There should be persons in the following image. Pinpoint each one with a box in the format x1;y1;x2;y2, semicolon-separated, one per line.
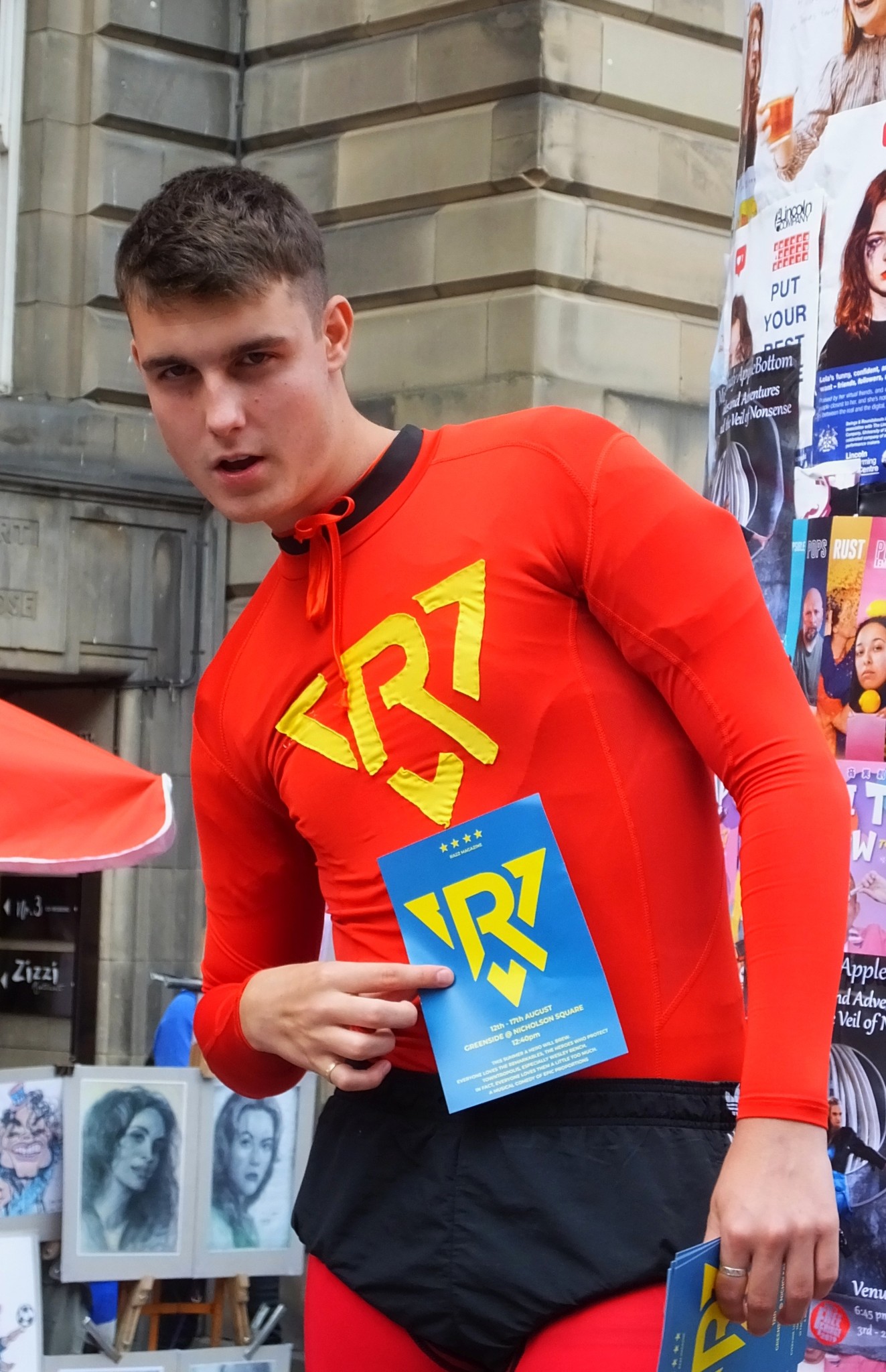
731;0;886;1372
115;166;853;1372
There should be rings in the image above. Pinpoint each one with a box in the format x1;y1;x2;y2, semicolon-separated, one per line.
719;1265;744;1276
324;1059;344;1083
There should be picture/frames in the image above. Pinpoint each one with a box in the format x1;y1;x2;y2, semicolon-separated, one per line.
46;1341;295;1372
59;1064;202;1285
191;1071;318;1279
0;1062;66;1372
